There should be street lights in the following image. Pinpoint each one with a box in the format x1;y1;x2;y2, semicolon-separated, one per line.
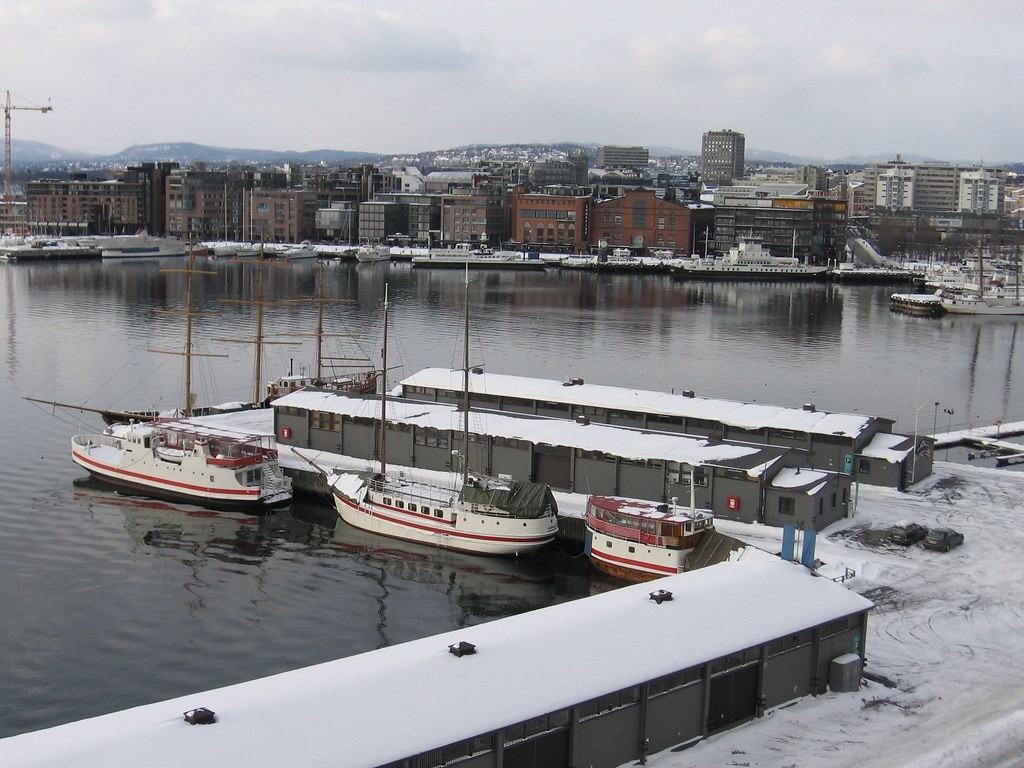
940;231;984;298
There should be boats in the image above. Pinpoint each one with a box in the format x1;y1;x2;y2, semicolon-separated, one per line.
340;248;360;261
236;250;261;256
71;431;294;512
309;244;360;257
899;247;1024;296
560;255;594;269
327;261;562;557
583;496;715;584
669;228;829;282
99;224;404;425
939;292;1024;315
186;239;208;255
212;182;238;256
273;245;293;258
831;263;933;284
95;229;186;257
284;240;318;258
411;243;545;270
355;238;392;261
890;293;941;316
590;238;692;272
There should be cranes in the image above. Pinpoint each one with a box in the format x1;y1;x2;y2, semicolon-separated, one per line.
0;89;54;219
